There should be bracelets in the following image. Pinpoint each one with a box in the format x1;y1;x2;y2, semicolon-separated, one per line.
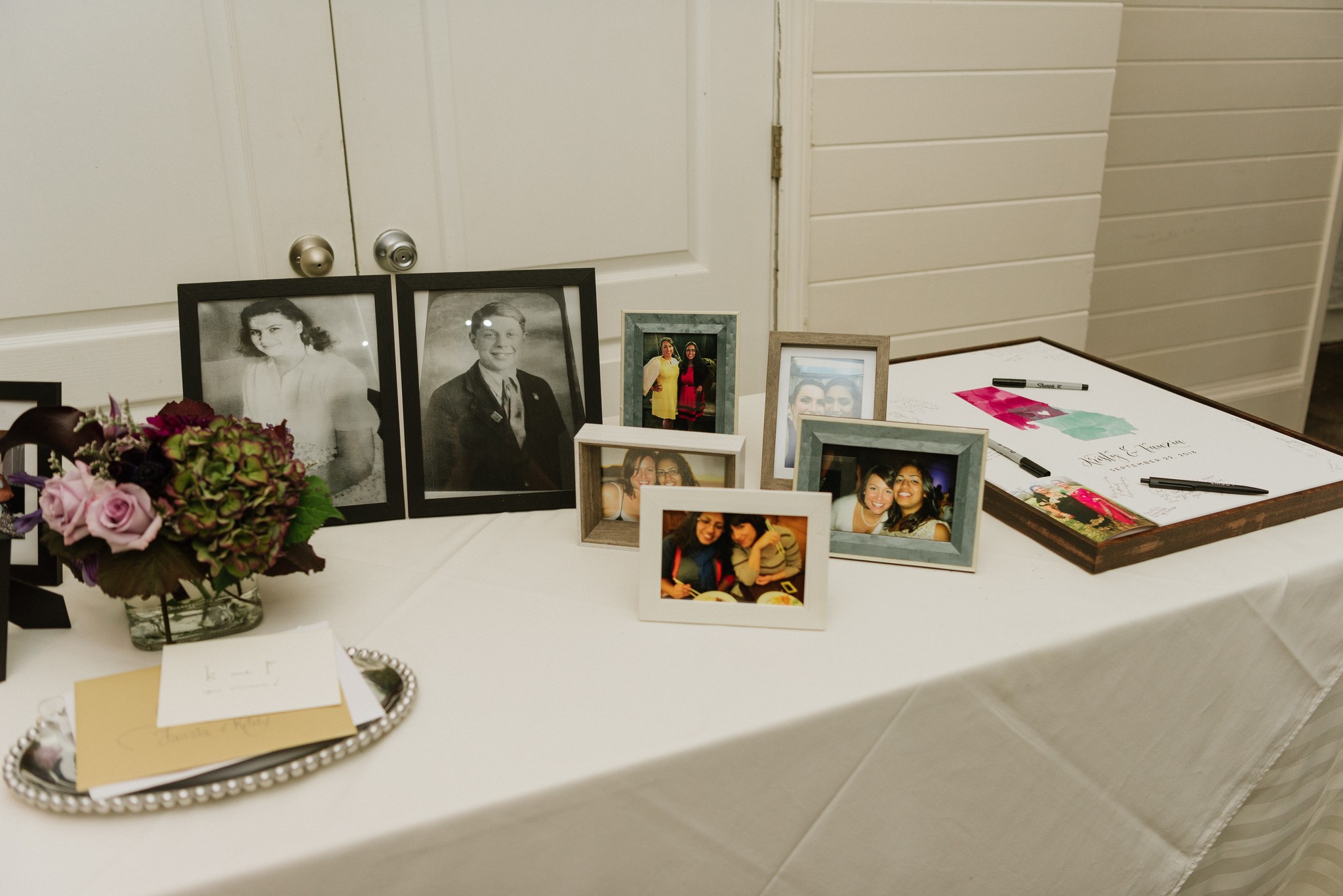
1058;512;1062;515
1063;513;1070;518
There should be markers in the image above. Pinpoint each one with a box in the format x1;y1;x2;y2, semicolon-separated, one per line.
987;437;1051;478
991;378;1089;391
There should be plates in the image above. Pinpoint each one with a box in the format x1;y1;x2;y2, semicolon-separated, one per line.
692;591;738;603
756;591;803;606
780;581;798;594
4;647;418;816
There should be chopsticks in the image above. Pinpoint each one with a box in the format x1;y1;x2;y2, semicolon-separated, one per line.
765;518;785;556
672;577;701;598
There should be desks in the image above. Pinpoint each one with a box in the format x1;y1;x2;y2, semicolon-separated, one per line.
1;387;1343;896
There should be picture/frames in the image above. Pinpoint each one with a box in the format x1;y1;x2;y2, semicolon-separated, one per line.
886;335;1343;581
177;273;407;528
759;331;892;490
635;481;833;635
793;421;993;574
0;375;64;590
574;421;748;549
617;308;741;441
392;264;607;522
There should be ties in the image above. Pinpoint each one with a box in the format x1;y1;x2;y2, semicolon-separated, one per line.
500;377;526;448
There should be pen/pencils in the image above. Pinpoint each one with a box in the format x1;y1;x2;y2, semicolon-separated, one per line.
1140;476;1269;495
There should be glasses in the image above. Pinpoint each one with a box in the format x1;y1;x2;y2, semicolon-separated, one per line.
657;468;682;478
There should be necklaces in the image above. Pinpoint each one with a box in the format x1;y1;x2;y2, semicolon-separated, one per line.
687;364;693;368
1064;485;1078;496
861;506;886;526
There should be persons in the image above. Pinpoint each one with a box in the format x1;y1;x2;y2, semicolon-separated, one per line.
422;301;576;491
825;376;862;419
921;470;954;523
879;460;951;542
237;298;386;505
820;445;861;502
601;448;656;522
643;337;679;430
785;379;826;468
655;452;700;486
1051;480;1138;526
678;342;710;431
661;512;735;599
724;514;801;587
1;472;14;566
1029;485;1126;534
829;465;894;535
